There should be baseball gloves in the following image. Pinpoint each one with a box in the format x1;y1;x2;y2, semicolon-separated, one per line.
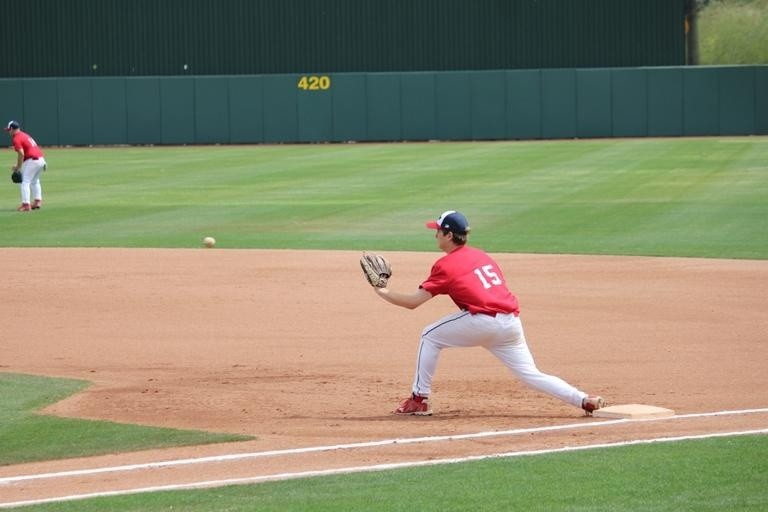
12;170;22;183
361;255;393;288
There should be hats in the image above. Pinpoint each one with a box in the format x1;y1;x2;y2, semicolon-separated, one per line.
3;121;19;131
426;210;470;235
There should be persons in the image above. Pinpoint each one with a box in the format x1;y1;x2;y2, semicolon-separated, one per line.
358;209;603;418
2;120;47;211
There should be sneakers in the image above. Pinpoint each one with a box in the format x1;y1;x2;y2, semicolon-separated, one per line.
394;392;432;416
17;204;31;211
583;394;606;412
32;201;40;209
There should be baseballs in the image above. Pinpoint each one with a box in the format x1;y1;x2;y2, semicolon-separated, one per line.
204;237;215;246
